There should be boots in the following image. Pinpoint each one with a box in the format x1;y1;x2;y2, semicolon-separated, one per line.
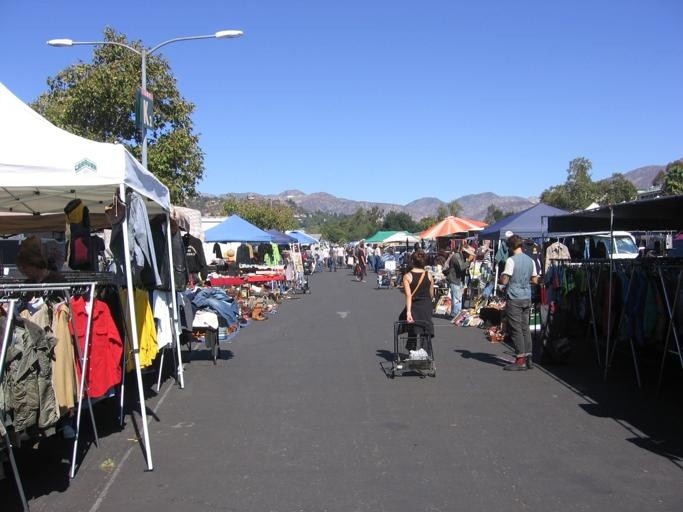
252;307;266;320
504;356;533;371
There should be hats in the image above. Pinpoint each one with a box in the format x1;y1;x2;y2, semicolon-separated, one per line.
462;245;476;257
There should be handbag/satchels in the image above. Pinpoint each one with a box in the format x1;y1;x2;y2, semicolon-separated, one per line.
398;306;407;334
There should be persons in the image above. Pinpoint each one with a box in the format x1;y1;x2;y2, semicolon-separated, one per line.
281;239;494;319
15;246;69;297
402;250;435;359
498;234;538;370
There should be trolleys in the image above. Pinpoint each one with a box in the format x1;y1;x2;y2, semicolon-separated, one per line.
376;268;392;289
391;319;435;378
300;268;311;295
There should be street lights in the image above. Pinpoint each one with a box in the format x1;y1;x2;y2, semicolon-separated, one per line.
247;193;298;218
45;28;245;171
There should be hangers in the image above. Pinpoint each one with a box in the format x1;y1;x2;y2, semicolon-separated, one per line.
547;237;683;278
0;189;199;309
